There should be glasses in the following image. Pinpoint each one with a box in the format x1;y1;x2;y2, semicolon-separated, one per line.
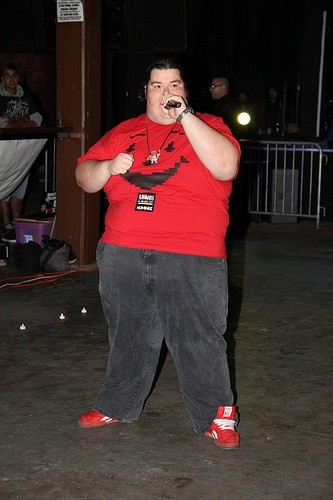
209;84;224;90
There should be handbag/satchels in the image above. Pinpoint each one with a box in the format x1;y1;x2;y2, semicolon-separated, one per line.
13;237;72;274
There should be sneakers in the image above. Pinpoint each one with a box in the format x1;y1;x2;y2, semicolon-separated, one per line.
77;410;118;428
203;405;240;449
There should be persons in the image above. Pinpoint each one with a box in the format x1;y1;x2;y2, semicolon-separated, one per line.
238;91;247;110
0;62;37;243
209;77;238;135
266;88;281;111
74;59;240;448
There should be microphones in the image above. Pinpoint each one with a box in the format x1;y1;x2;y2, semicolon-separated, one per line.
164;100;182;109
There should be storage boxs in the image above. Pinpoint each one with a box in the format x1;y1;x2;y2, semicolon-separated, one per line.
13;218;55;247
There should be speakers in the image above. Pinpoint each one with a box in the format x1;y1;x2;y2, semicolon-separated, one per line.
0;0;187;132
271;168;299;223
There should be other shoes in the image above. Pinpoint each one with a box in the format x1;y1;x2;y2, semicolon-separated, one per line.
0;259;7;267
1;229;17;243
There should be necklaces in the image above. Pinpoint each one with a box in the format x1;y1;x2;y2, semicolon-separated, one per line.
145;115;178;164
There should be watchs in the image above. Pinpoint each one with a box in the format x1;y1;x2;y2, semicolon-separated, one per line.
176;105;194;123
